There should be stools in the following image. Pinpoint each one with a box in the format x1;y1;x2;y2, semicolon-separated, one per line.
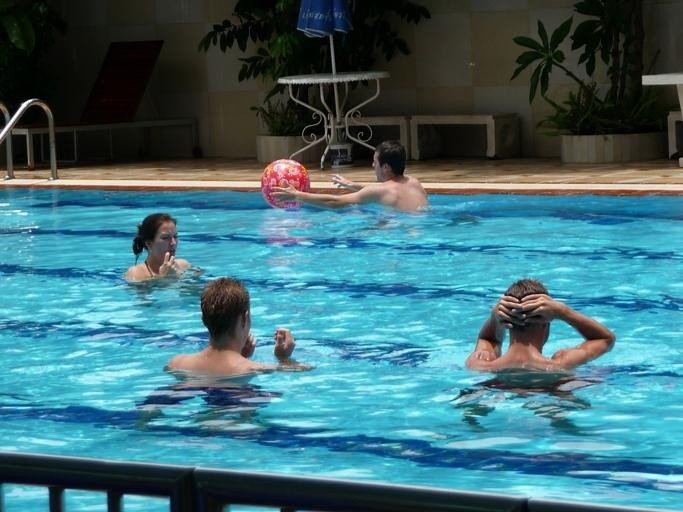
330;111;523;159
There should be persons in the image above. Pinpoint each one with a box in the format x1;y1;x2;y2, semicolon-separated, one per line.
269;140;428;211
132;276;307;436
462;278;615;433
124;212;190;283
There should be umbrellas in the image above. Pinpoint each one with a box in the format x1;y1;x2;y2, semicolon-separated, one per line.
296;0;354;124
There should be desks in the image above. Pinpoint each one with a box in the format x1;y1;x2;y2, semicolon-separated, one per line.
640;71;682;169
277;72;390;170
